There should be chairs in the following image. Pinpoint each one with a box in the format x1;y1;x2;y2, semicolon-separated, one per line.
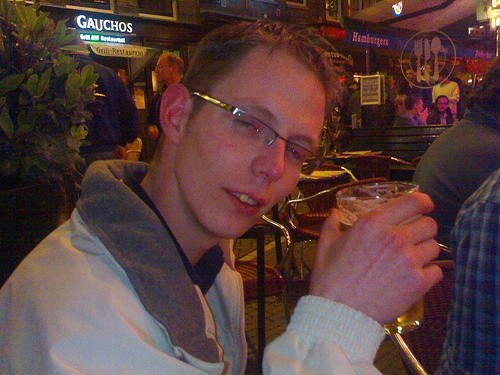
236;152;454;375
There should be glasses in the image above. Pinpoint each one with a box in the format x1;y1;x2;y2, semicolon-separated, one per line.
190;92;317;176
155;63;172;70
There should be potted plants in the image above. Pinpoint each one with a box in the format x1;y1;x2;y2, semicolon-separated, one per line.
0;0;108;283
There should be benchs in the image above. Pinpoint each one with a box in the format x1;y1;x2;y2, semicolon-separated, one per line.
349;124;452;171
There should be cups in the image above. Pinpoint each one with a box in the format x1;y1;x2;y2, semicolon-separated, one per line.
336;182;423;334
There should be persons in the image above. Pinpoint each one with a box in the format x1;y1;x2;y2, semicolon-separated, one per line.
343;69;462;128
64;30;189;166
414;55;500;374
0;18;445;375
